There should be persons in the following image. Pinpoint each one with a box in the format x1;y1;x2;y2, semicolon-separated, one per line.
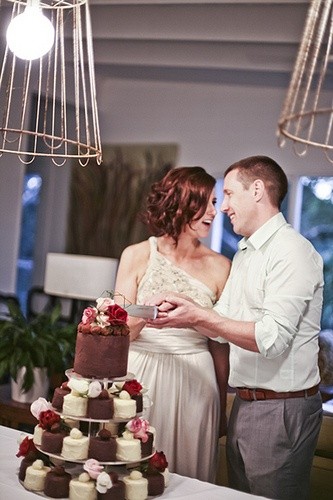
145;156;323;500
114;166;232;486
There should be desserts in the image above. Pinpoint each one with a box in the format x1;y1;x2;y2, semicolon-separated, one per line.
18;457;165;500
33;418;156;461
51;381;144;420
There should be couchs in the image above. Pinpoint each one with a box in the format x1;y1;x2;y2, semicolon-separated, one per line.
218;329;333;500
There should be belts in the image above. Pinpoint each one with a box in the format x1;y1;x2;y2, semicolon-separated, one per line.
236;384;319;400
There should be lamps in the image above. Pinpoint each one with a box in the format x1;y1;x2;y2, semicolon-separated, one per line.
0;0;103;167
44;250;119;302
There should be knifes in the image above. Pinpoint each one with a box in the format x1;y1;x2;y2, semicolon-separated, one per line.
119;304;158;320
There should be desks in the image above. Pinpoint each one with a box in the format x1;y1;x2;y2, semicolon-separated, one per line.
0;419;273;500
0;370;66;432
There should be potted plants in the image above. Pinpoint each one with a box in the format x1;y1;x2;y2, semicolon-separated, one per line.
0;296;77;402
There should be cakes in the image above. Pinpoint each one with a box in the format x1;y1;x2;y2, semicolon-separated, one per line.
72;322;130;378
